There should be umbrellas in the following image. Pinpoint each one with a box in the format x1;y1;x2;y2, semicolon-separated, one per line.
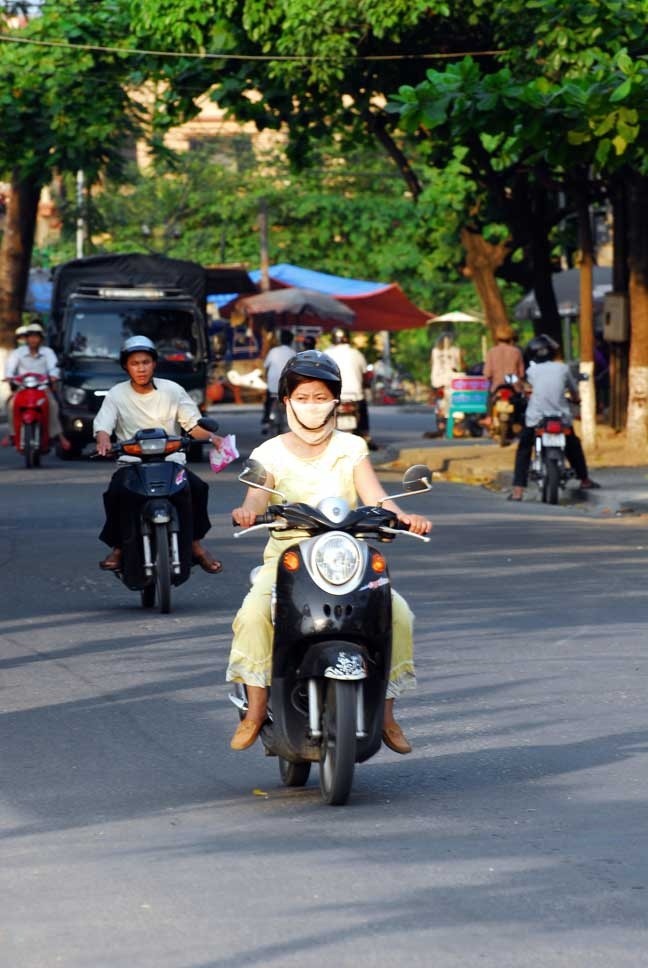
426;310;481;325
237;288;355;325
514;265;613;319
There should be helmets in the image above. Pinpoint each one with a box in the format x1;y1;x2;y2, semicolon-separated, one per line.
331;325;350;345
119;335;158;370
528;334;561;363
27;323;45;338
496;326;514;341
14;325;27;339
279;349;340;397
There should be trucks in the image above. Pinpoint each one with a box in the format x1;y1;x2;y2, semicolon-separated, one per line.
44;251;231;467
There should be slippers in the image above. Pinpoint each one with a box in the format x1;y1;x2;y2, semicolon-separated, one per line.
581;482;600;488
507;494;522;501
193;551;223;574
99;553;122;569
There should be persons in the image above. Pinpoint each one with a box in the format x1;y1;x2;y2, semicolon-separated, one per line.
226;348;432;754
3;323;72;449
92;334;225;574
483;326;605;502
259;329;378;451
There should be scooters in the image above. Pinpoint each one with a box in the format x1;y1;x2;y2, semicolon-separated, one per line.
231;457;433;812
485;379;527;448
260;369;374;444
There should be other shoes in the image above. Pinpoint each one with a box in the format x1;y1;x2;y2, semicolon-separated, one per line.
0;433;11;447
383;724;411;754
231;714;269;751
60;438;72;451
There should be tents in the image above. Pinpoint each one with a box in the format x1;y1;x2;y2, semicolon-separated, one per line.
21;263;433;332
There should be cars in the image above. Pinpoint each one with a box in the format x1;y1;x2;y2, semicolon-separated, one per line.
210;319;257;360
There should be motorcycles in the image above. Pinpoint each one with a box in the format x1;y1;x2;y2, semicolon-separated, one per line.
1;372;63;469
504;367;590;505
86;416;219;614
